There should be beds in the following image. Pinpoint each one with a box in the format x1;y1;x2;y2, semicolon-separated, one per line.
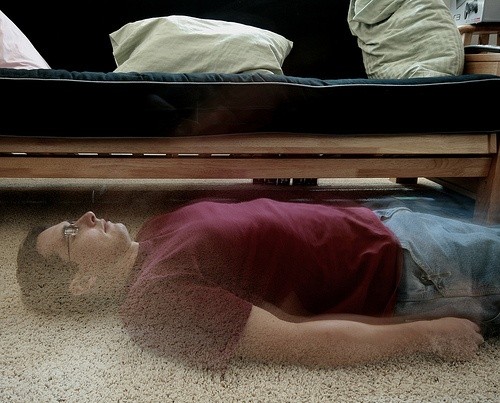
1;1;500;233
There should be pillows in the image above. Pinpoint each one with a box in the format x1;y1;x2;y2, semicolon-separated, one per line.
346;1;466;84
106;15;294;82
0;9;51;71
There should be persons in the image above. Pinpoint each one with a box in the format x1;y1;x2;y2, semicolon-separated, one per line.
16;194;499;369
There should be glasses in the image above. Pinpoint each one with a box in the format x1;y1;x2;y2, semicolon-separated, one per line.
63;218;79;263
